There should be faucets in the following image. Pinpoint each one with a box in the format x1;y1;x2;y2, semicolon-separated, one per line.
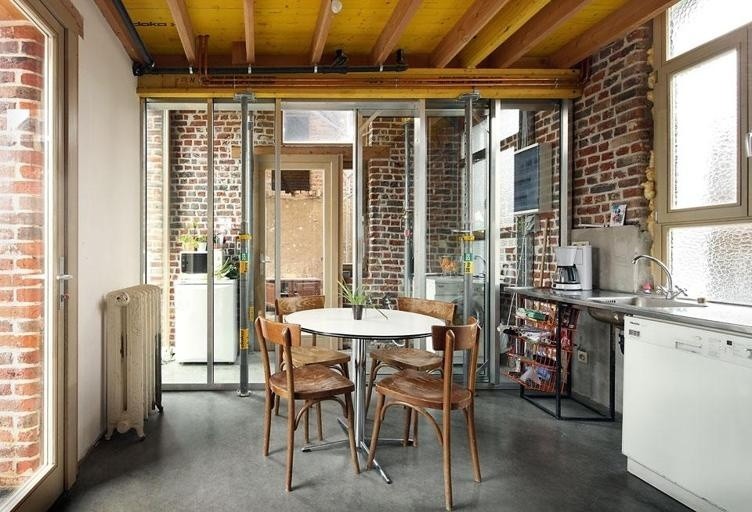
631;252;690;300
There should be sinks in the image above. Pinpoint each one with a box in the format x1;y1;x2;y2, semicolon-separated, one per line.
584;292;710;310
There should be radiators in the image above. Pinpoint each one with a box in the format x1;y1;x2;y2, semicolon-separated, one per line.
103;283;163;442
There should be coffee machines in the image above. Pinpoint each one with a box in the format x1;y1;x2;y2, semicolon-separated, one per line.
551;241;593;292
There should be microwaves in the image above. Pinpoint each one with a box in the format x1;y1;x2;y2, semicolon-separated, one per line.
178;251;223;276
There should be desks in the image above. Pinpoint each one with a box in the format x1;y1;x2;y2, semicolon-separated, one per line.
285;307;447;486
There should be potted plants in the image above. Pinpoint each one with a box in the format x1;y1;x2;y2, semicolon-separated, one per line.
175;234;208;251
335;275;389;321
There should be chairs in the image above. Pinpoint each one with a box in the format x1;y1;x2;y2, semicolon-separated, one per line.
364;295;459;446
365;315;485;508
273;296;352;416
254;311;361;491
